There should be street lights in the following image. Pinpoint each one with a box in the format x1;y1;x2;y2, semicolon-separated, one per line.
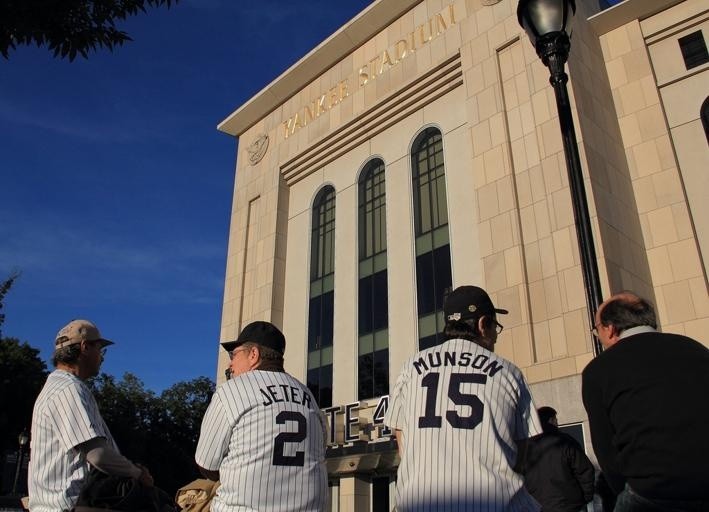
5;425;30;496
516;1;612;364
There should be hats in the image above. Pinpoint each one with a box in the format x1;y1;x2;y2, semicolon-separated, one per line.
220;320;286;353
54;318;115;352
443;285;509;323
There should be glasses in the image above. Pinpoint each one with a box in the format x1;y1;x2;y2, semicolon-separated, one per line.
483;315;504;334
591;322;603;336
84;347;108;358
227;348;250;361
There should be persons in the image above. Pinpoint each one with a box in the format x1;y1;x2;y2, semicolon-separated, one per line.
194;320;333;511
27;319;176;511
513;405;596;512
382;285;547;512
580;289;709;512
